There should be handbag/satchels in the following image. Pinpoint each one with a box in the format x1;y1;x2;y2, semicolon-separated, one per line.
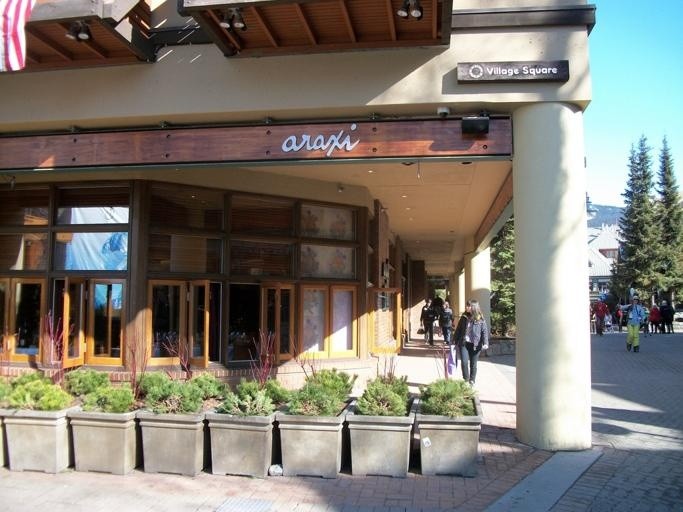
438;330;443;337
417;329;425;334
447;343;458;374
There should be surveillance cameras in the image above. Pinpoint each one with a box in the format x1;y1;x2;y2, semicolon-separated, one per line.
437;107;452;123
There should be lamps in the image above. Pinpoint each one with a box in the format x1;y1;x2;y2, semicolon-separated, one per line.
219;7;247;31
66;20;94;42
396;0;423;20
462;112;489;134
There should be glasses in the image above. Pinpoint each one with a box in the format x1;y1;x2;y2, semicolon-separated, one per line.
633;299;638;300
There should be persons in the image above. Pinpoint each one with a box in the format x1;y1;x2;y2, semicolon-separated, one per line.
439;301;455;345
420;297;438;347
452;300;489;386
590;293;675;352
432;295;444;320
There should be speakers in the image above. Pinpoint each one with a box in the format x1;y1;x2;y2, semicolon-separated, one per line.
462;117;489;135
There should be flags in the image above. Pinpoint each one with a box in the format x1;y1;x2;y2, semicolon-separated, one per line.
0;0;36;72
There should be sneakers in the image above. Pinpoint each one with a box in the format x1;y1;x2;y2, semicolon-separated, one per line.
634;346;639;352
627;343;631;351
